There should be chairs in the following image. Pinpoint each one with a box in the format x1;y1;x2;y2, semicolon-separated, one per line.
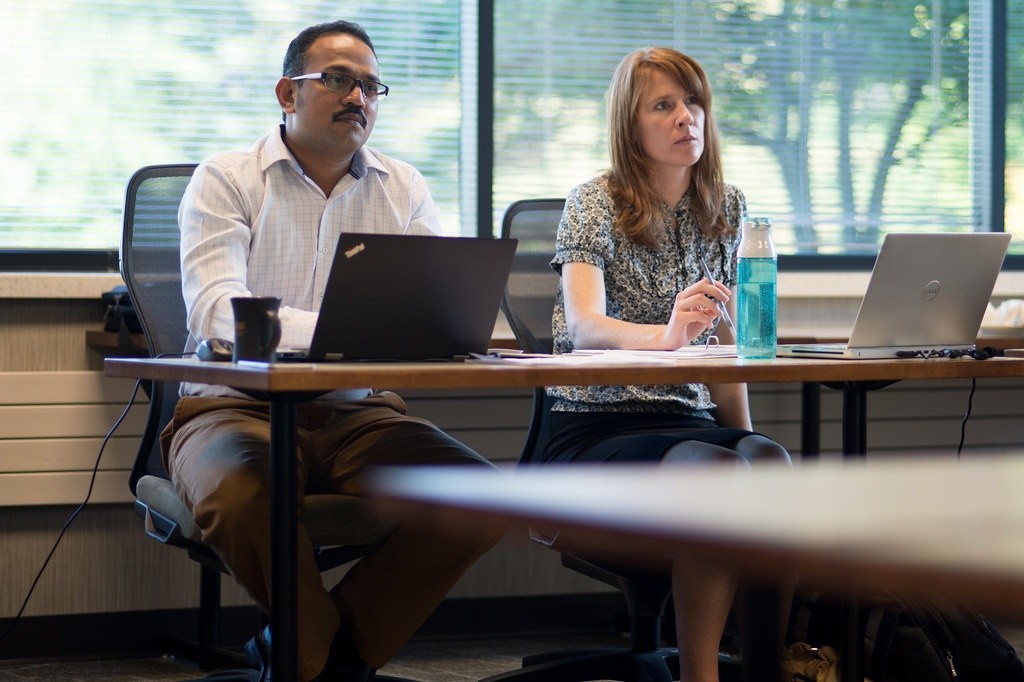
480;197;744;682
124;165;421;682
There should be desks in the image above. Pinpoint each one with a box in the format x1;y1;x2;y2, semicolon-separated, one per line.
372;445;1024;619
104;339;1024;682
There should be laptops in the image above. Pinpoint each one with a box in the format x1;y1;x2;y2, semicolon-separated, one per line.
274;233;518;362
773;232;1013;360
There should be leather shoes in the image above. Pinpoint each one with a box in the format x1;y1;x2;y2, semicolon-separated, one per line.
323;624;376;682
244;625;272;682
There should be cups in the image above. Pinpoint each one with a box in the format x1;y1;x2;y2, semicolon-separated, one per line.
229;296;283;364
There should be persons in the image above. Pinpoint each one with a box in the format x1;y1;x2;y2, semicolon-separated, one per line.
163;19;518;682
522;45;798;682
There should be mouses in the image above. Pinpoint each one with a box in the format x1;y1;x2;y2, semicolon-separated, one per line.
196;339;235;362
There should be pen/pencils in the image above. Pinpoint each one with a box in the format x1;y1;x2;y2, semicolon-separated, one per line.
696;253;734;329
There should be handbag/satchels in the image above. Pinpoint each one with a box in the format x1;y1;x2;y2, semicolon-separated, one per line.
734;587;1024;682
102;292;144;356
782;643;872;682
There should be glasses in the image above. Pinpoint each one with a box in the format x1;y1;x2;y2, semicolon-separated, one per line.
291;72;389;101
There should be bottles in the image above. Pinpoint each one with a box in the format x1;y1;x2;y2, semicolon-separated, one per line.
736;216;779;360
